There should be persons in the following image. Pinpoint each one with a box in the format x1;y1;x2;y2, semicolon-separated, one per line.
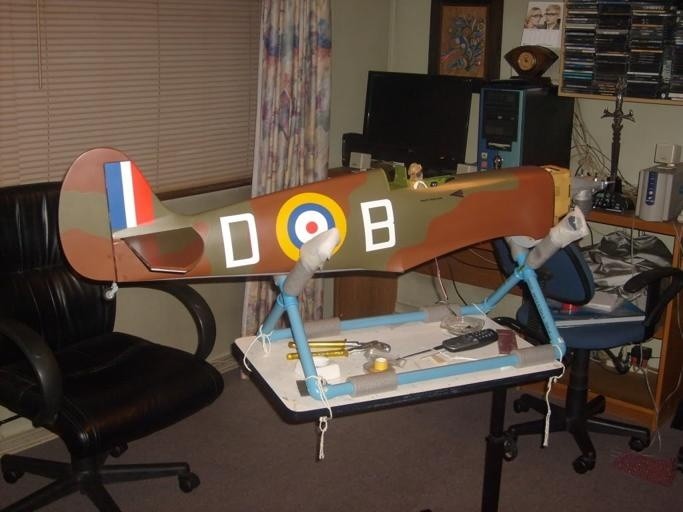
545;4;561;30
525;7;545;28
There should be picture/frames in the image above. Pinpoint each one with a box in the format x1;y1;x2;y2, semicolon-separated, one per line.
559;0;683;108
428;1;504;91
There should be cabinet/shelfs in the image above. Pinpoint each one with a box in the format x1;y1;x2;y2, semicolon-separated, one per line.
325;162;682;432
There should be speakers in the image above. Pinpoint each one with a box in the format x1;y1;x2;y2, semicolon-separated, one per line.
634;161;683;223
341;133;363;168
653;143;680;170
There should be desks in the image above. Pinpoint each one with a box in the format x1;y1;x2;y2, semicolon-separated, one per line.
234;313;565;512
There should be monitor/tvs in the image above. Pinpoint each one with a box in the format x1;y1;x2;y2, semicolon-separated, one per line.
361;71;474;179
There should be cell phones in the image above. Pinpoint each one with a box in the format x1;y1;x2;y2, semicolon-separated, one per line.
442;328;498;352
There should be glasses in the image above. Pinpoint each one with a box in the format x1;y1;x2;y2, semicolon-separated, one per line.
530;13;542;18
543;11;558;17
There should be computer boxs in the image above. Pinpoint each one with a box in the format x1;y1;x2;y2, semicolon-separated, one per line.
477;87;575;173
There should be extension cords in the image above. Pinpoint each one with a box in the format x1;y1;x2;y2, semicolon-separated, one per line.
605;357;648;375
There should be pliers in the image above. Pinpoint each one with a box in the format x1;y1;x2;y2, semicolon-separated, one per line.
286;338;391;360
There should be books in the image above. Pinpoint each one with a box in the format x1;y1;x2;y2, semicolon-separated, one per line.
562;0;683;104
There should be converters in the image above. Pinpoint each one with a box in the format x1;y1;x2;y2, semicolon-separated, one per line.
631;345;652;360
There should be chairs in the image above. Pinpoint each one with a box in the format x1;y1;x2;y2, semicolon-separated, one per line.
0;181;223;511
491;236;682;474
